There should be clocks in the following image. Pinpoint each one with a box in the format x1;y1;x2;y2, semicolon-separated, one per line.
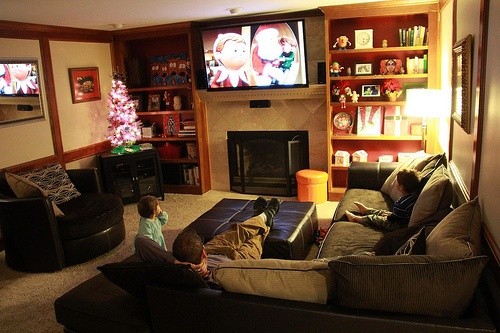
354;28;373;49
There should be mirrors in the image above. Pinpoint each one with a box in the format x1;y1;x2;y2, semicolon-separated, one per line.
0;58;47;128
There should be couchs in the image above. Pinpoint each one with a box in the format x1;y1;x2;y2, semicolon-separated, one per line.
147;160;500;333
0;168;125;271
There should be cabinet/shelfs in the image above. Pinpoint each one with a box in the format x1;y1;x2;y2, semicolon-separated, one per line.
318;1;440;201
115;20;208;195
96;145;164;202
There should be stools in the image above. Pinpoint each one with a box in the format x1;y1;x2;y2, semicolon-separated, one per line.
296;169;328;204
54;251;171;333
175;197;319;260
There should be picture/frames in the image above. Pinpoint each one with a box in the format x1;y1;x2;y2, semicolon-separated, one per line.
451;34;473;134
68;67;101;105
148;95;161;112
354;62;374;77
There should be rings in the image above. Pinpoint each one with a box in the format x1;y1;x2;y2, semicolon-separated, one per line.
198;268;201;272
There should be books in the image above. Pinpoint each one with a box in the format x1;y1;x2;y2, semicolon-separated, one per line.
399;26;427;75
178;121;197;138
184;165;200;186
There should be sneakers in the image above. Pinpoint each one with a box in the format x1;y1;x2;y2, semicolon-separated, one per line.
264;198;280;227
253;196;267;217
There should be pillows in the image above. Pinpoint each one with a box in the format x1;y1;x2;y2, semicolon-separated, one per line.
408;164;454;226
96;262;208;297
426;196;482;260
395;226;427;255
380;150;435;199
2;170;63;217
19;162;82;204
373;224;424;253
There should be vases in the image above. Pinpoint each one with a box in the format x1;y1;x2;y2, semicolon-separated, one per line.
385;91;402;102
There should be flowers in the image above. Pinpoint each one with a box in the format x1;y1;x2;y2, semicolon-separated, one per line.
381;78;402;94
107;79;141;149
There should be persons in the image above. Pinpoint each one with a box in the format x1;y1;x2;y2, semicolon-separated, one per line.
328;34;352;77
135;196;280;286
345;170;418;231
0;63;39;95
364;88;373;95
203;23;300;89
